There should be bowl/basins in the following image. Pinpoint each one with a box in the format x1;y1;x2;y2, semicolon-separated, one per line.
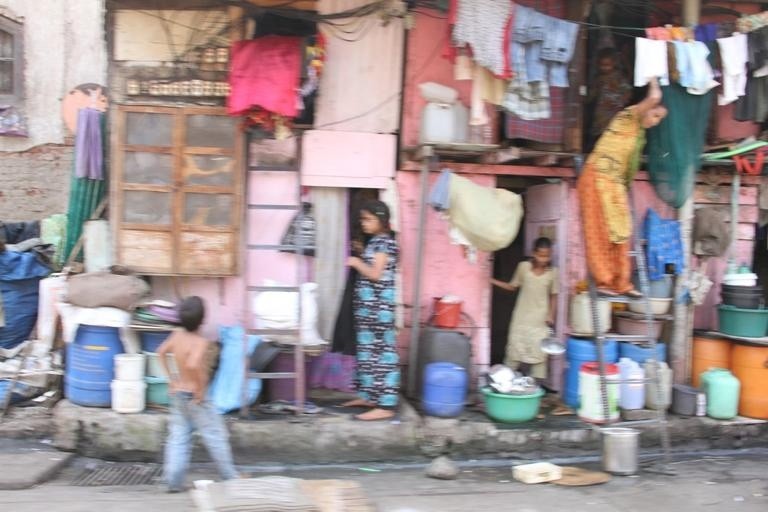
632;271;675;298
627;297;674;315
480;387;546;424
135;328;176;406
717;304;768;338
614;314;665;338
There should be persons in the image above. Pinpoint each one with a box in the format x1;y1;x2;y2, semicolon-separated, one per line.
156;295;251;494
345;201;400;421
579;75;668;299
487;239;559;389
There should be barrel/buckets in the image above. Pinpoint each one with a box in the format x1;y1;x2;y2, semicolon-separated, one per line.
417;329;471;397
569;290;612;335
110;380;146;413
599;428;641;475
113;354;147;379
699;368;741;421
577;360;621;425
64;325;125;410
730;341;768;421
692;332;731;390
618;339;667;363
432;296;464;328
644;360;673;411
420;102;467;141
616;357;644;409
421;361;468;419
562;339;619;407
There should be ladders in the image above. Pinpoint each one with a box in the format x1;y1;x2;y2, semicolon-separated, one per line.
573;156;672;464
235;129;306;420
